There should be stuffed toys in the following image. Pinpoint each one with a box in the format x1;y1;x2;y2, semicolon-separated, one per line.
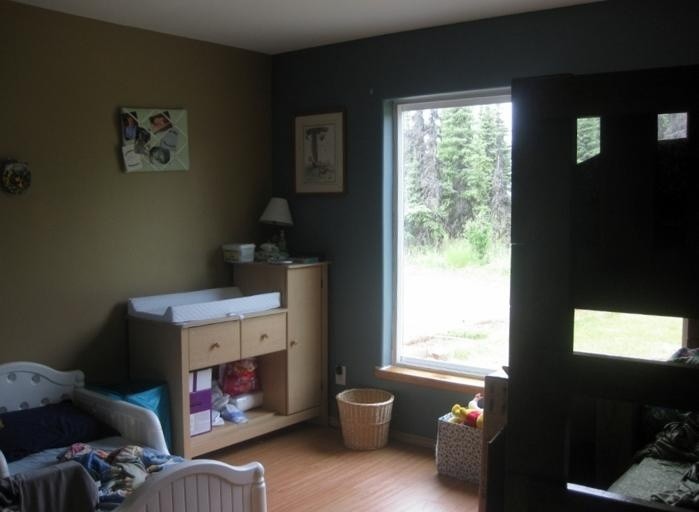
448;392;484;430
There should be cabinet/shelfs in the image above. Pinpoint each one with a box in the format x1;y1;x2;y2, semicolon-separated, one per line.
231;262;327;424
127;309;287;373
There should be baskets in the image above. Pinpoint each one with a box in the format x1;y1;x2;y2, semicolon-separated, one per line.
336;388;395;450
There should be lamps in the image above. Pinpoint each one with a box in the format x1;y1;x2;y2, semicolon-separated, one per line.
258;197;295;250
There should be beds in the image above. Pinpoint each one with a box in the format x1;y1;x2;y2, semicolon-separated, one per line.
1;362;268;512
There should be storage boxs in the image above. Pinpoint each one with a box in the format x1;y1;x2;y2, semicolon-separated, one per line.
435;412;485;486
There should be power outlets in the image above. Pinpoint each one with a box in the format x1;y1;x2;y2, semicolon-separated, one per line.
335;365;346;386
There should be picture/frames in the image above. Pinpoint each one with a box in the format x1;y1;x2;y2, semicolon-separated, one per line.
289;105;348;199
